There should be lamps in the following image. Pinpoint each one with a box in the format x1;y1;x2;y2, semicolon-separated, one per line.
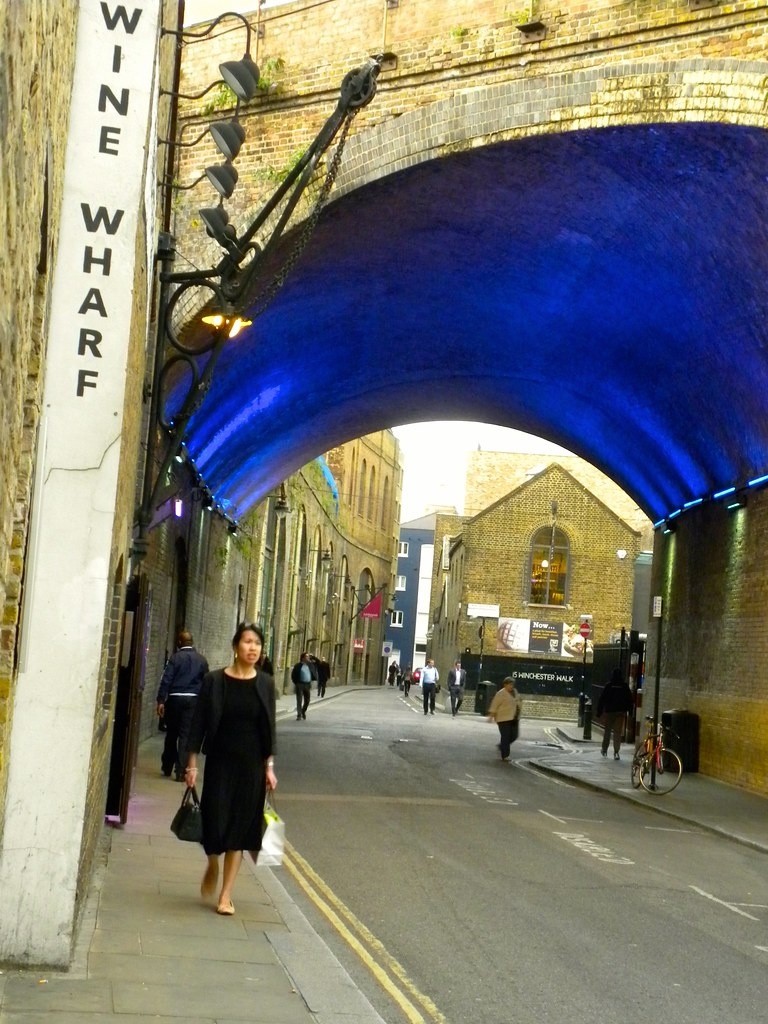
332;574;352;587
201;286;256;338
229;527;239;535
156;174;232;236
309;549;332;570
175;449;186;463
206;499;215;510
157;79;245;162
388;592;399;605
158;12;260;104
266;495;291;520
157;127;237;199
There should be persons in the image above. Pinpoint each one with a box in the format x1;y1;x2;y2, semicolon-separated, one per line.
291;653;322;720
419;659;439;715
395;666;402;686
401;666;413;697
317;657;330;698
447;661;465;716
255;650;273;675
596;668;634;759
487;677;523;761
387;663;396;686
184;622;278;914
156;632;209;782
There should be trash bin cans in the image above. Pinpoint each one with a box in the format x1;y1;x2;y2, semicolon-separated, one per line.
661;708;701;773
477;680;496;717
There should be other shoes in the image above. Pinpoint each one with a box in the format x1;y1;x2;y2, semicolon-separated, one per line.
614;752;619;760
321;695;323;697
318;693;320;696
497;744;501;752
302;711;306;719
176;773;184;781
504;757;511;762
430;712;434;714
217;899;234;915
453;714;456;717
161;770;171;777
202;880;216;897
601;749;607;756
297;715;301;720
424;711;427;715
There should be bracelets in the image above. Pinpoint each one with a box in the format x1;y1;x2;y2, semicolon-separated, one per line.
267;762;273;767
186;767;198;772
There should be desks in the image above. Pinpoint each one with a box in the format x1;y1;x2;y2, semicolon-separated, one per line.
532;578;555;605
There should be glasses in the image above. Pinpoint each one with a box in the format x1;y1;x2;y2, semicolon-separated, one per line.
244;623;261;629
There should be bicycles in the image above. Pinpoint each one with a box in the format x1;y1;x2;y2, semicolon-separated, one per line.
631;716;683;795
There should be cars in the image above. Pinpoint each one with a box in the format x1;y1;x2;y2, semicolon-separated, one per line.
413;668;423;682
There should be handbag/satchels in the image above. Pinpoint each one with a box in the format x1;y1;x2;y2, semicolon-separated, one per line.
400;685;404;691
170;787;202;842
515;706;521;720
435;684;439;693
248;787;286;868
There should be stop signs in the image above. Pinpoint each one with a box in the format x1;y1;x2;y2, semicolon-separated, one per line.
579;623;591;638
